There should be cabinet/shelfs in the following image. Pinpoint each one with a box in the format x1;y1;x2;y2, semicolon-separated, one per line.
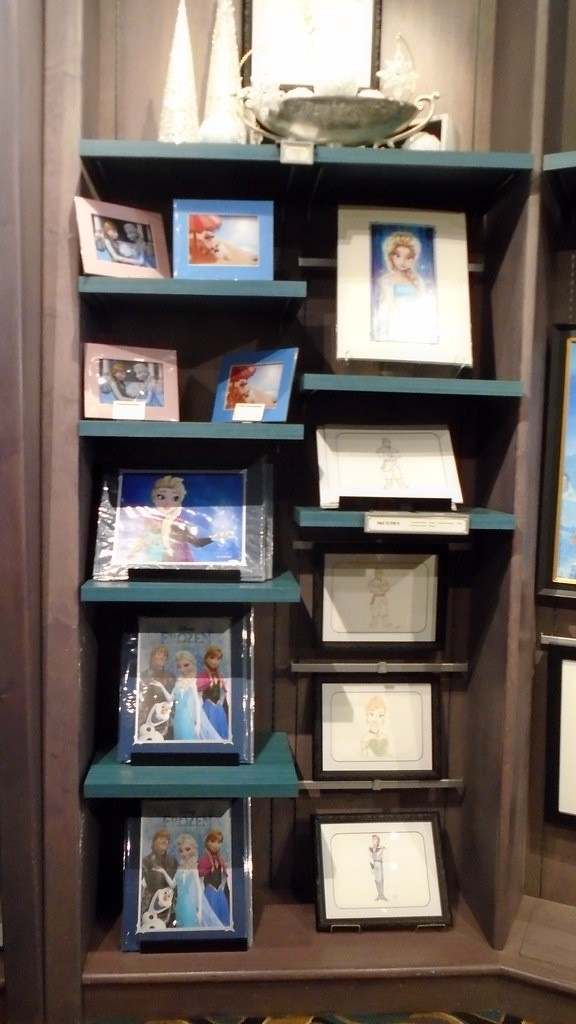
42;0;575;1024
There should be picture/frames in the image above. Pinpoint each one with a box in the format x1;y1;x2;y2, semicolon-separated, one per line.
536;326;575;603
72;196;477;950
544;644;575;828
